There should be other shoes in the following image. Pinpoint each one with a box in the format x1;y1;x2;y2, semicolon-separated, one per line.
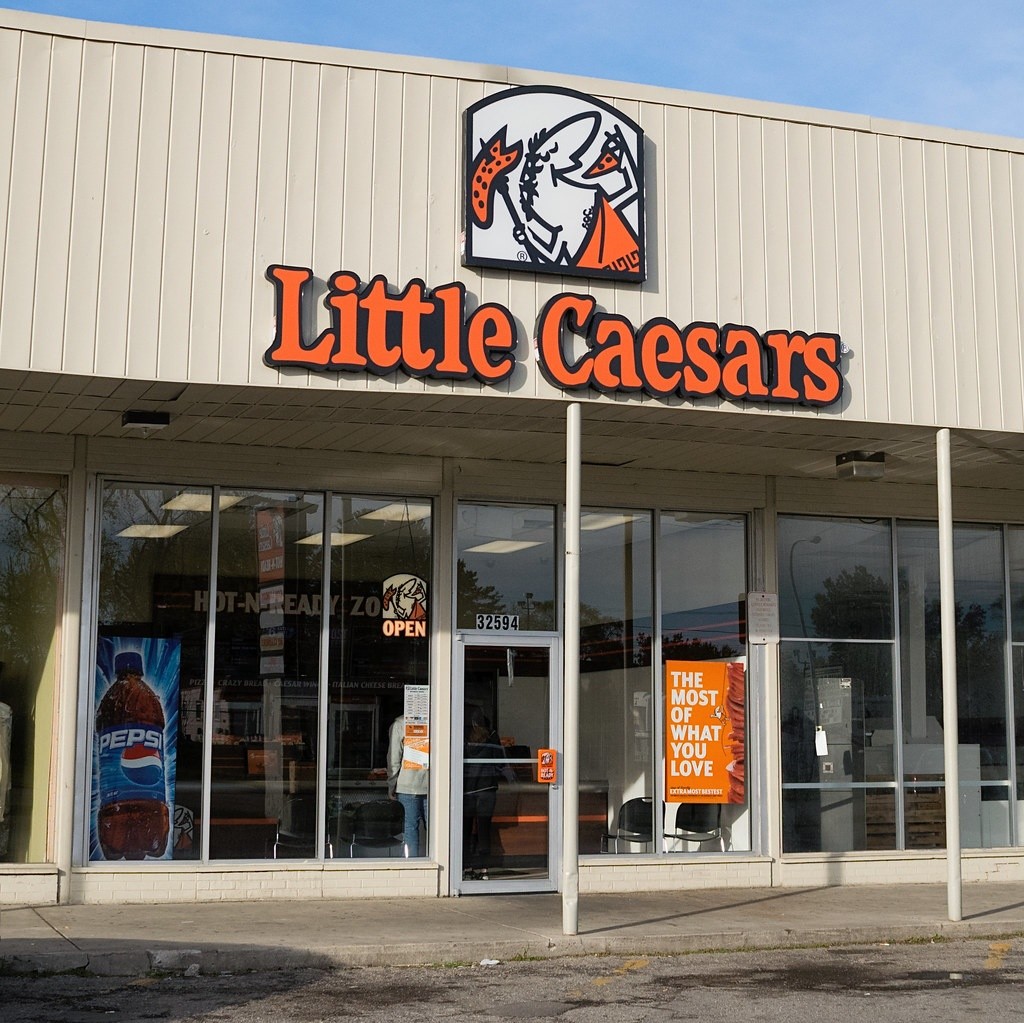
465;868;490;880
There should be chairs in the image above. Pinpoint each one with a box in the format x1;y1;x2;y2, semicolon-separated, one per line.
600;797;666;854
340;799;408;858
269;797;333;859
663;802;723;853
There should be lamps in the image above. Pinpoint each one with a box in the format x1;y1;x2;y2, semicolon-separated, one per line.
836;450;887;483
120;409;169;437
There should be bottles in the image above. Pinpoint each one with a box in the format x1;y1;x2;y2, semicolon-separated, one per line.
96;653;171;861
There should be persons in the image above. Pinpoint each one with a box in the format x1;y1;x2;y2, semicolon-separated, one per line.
462;703;505;880
386;712;431;858
